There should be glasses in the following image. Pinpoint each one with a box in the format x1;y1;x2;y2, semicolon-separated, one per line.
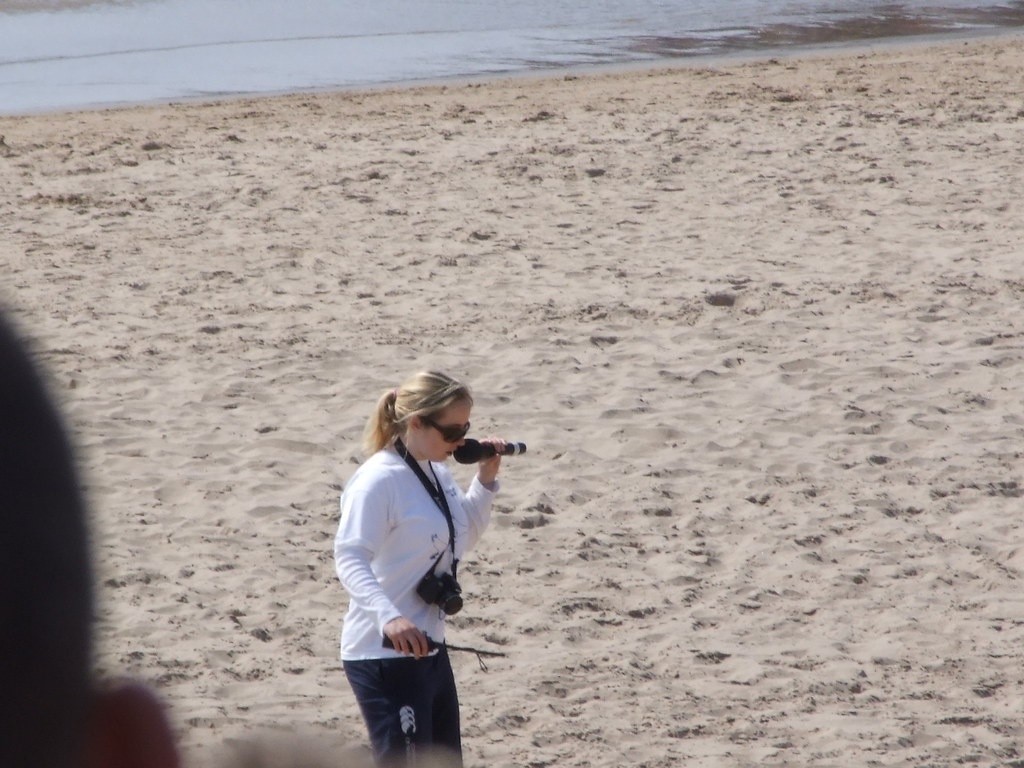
418;415;470;443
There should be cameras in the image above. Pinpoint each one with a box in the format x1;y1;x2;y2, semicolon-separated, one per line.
415;571;463;616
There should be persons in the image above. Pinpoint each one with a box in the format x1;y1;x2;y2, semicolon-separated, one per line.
0;313;181;768
335;367;506;768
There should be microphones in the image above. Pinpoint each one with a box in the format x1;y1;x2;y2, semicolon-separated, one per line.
453;438;526;465
